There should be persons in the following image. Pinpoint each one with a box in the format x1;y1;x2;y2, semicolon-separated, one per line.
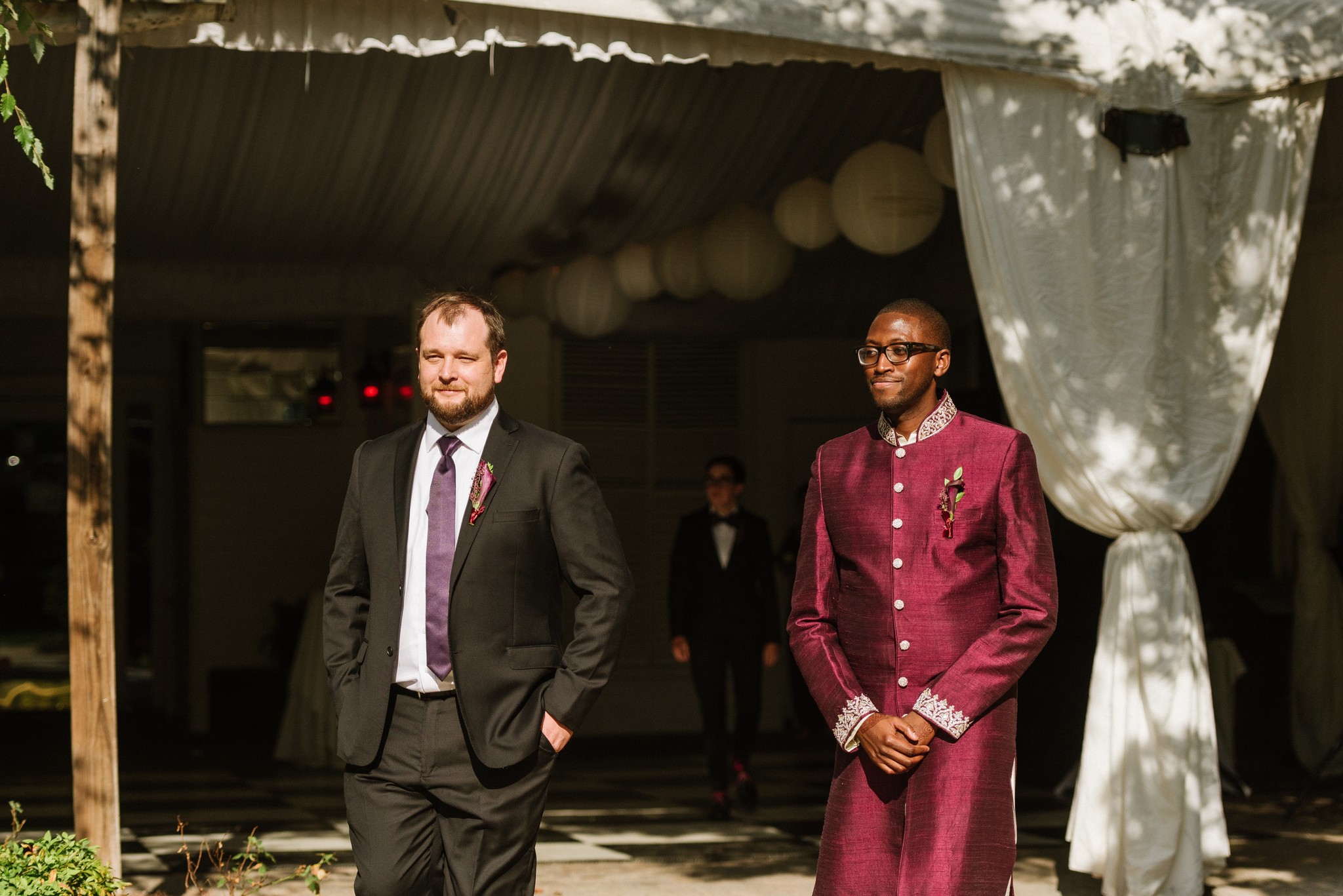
786;296;1064;896
318;290;641;895
666;452;780;824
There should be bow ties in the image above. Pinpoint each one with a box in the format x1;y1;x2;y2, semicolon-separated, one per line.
708;512;740;526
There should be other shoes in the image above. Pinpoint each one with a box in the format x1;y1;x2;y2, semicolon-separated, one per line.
735;770;758;812
709;799;732;822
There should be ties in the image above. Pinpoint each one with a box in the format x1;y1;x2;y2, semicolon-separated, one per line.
425;435;462;683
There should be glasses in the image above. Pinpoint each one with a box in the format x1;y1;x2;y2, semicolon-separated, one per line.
705;473;736;487
852;342;943;366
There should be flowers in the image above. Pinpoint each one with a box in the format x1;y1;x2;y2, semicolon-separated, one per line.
937;466;965;537
467;458;497;527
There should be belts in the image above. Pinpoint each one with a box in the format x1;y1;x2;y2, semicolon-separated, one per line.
391;683;457;700
